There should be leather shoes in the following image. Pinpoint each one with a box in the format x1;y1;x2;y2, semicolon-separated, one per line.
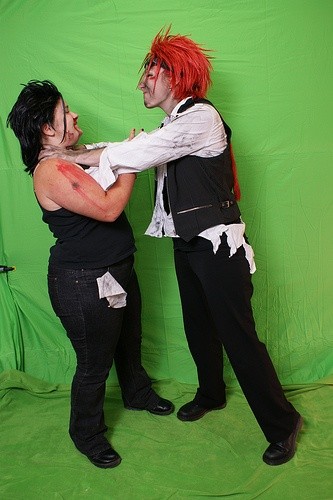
262;412;304;466
176;387;227;422
71;435;121;468
124;393;175;415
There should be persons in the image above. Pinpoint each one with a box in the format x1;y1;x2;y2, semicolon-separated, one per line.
7;79;175;468
38;25;303;467
